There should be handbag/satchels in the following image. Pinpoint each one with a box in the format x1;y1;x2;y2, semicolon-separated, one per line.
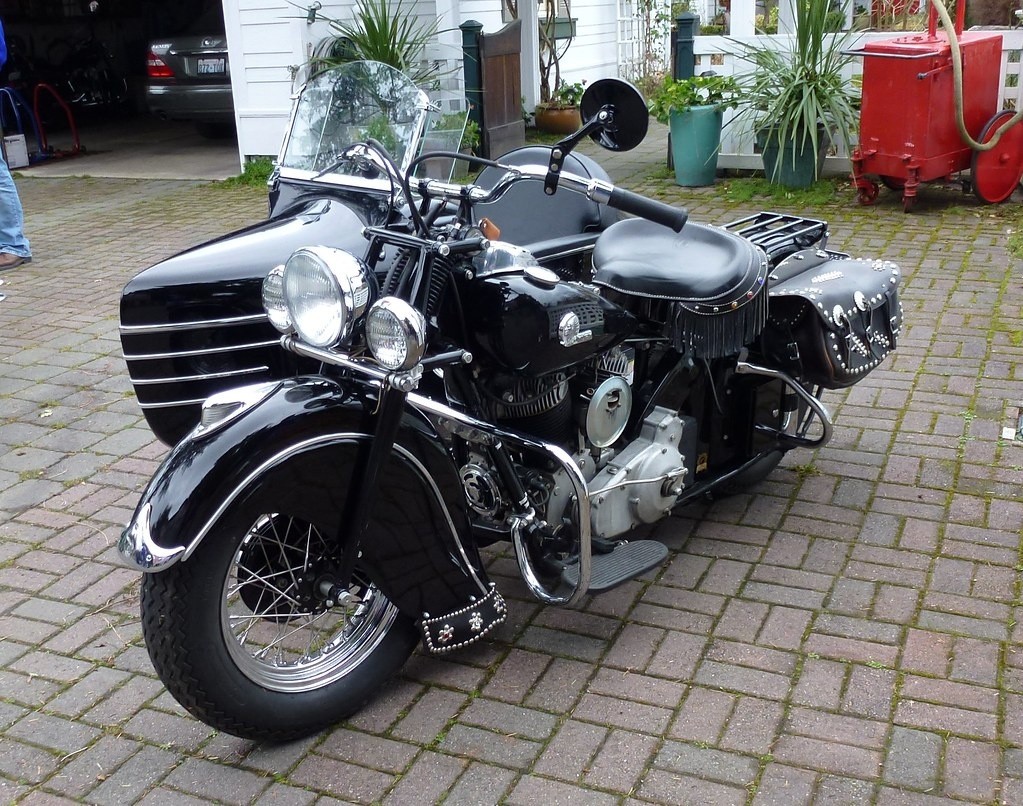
764;249;903;389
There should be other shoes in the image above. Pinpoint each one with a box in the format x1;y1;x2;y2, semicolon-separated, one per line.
0;253;32;272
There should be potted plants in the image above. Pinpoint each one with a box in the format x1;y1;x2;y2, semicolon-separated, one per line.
718;0;870;190
282;0;486;170
535;79;584;135
653;71;742;187
419;109;482;179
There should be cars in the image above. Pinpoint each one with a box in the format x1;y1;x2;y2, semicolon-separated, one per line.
145;0;239;141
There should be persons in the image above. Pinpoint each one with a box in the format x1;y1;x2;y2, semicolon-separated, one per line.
0;21;32;270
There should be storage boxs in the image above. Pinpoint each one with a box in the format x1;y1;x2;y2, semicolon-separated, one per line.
4;134;30;169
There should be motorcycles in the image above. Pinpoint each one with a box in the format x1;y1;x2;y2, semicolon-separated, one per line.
115;57;907;745
